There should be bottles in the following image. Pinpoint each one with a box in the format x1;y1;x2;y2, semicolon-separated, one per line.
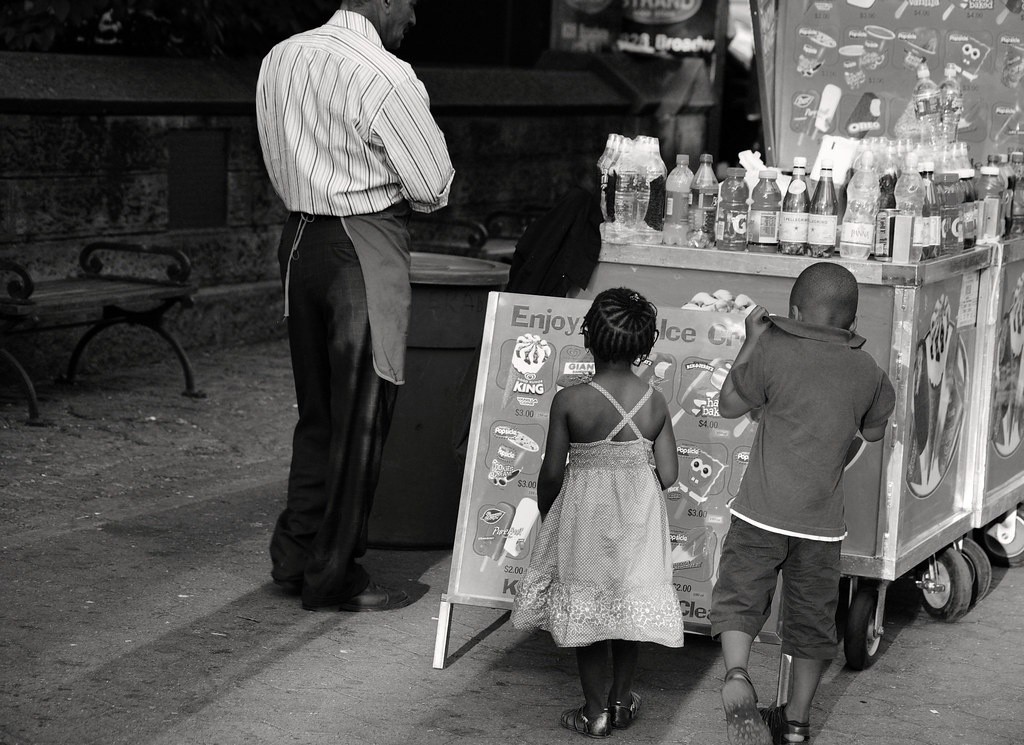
836;137;1024;264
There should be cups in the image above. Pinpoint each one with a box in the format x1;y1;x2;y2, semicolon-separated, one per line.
715;167;749;252
663;154;694;248
862;25;895;71
998;43;1024;89
686;154;719;250
780;157;810;257
486;429;540;490
902;41;934;74
747;171;782;254
838;43;867;91
939;67;962;143
795;31;836;78
915;61;945;144
808;161;838;258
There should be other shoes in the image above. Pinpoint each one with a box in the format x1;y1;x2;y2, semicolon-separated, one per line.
301;578;412;612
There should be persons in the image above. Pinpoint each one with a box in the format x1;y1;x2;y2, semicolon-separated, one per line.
535;288;679;740
710;261;897;745
255;0;455;613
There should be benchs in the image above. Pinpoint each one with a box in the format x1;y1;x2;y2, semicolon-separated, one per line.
400;209;546;267
0;241;208;426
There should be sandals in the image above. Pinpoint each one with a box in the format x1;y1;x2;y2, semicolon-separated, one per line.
560;701;612;738
608;689;642;728
756;702;811;745
719;667;775;745
